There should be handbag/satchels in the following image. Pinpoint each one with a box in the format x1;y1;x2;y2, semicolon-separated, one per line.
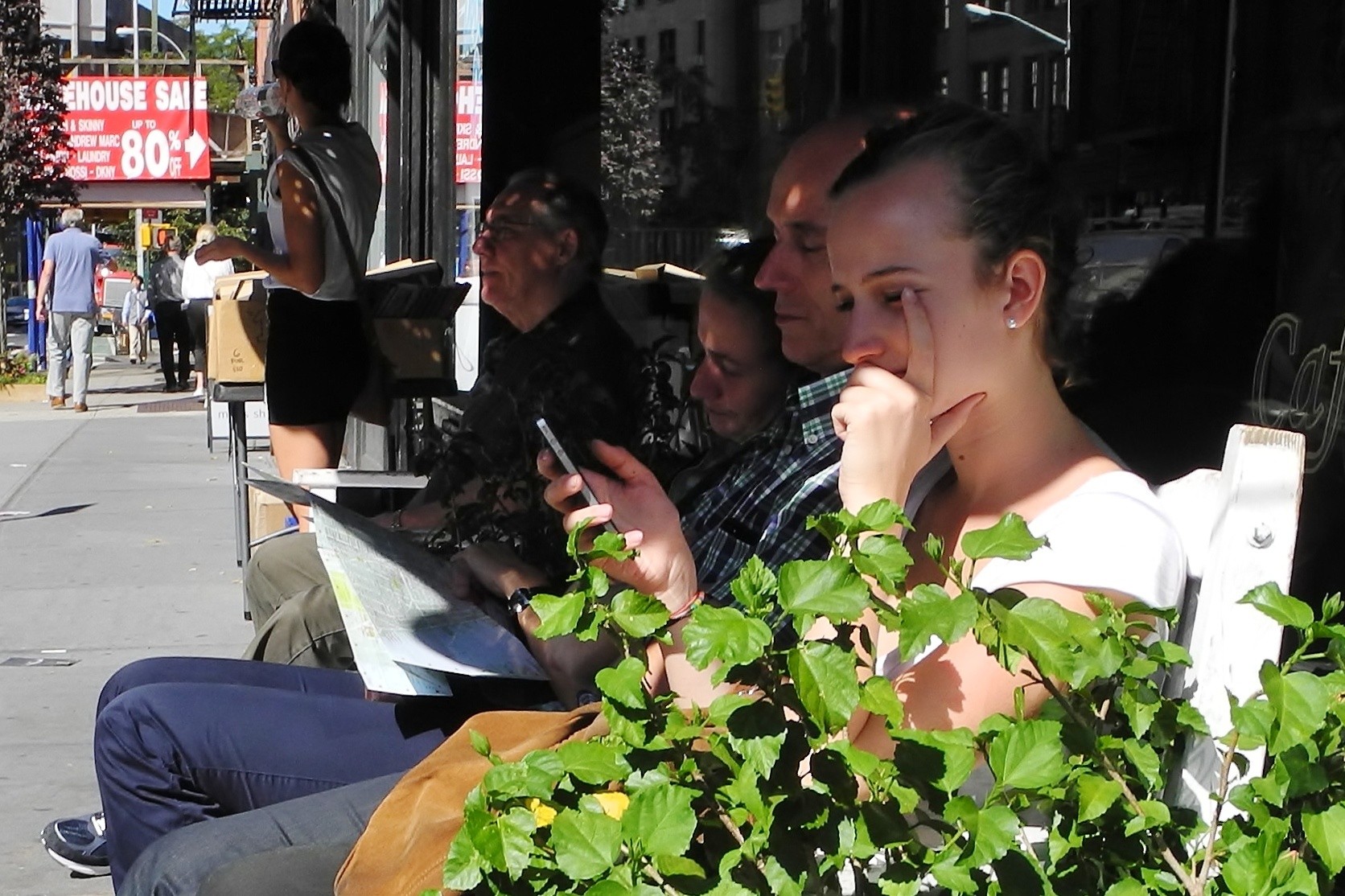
350;314;393;429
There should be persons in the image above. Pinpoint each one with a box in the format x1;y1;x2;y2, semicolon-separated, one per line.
181;223;235;397
121;273;154;364
41;228;805;875
34;207;118;414
194;16;382;533
147;235;192;393
115;77;1191;896
247;152;648;669
94;93;934;883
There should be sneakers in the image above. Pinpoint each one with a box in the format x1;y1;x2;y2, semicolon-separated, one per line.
42;813;111;878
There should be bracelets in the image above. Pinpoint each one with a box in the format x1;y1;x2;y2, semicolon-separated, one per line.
389;508;403;530
667;589;707;622
507;586;560;615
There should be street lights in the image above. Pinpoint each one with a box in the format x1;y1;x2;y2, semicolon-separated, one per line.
114;25;212;225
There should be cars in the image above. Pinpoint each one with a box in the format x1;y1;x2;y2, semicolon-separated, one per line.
5;296;30;325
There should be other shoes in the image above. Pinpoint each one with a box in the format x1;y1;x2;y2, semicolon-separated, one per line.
130;359;136;363
179;379;188;388
163;382;174;391
74;402;87;412
140;360;146;364
51;396;65;410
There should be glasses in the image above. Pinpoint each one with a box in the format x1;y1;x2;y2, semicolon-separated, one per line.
474;220;557;241
271;59;285;80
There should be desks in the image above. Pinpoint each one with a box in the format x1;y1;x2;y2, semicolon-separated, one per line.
214;380;464;621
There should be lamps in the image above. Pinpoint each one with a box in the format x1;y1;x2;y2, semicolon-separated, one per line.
964;4;1067;47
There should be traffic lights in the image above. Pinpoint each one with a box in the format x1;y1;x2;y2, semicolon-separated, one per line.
152;227;178;251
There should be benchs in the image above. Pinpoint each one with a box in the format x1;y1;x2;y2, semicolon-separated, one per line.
292;333;1306;896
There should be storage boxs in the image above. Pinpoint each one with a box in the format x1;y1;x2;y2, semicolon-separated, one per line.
210;383;271;438
208;282;471;378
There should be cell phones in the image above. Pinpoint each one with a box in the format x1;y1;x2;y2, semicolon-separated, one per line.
527;412;620;535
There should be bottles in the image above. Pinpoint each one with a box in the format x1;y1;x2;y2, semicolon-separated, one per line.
235;83;284;120
